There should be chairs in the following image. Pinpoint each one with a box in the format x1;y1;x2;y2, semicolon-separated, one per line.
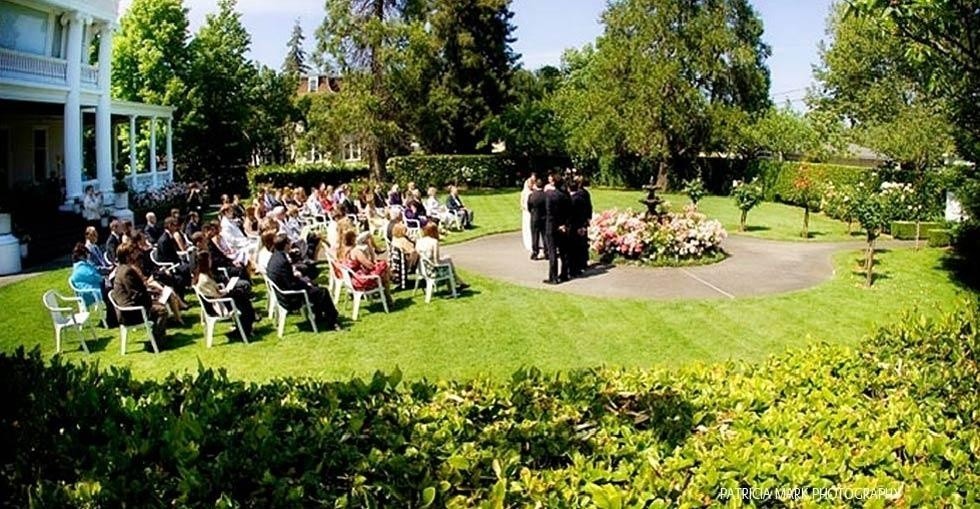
43;194;473;355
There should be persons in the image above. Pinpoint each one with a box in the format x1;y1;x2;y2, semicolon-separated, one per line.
520;170;592;284
47;171;60;207
